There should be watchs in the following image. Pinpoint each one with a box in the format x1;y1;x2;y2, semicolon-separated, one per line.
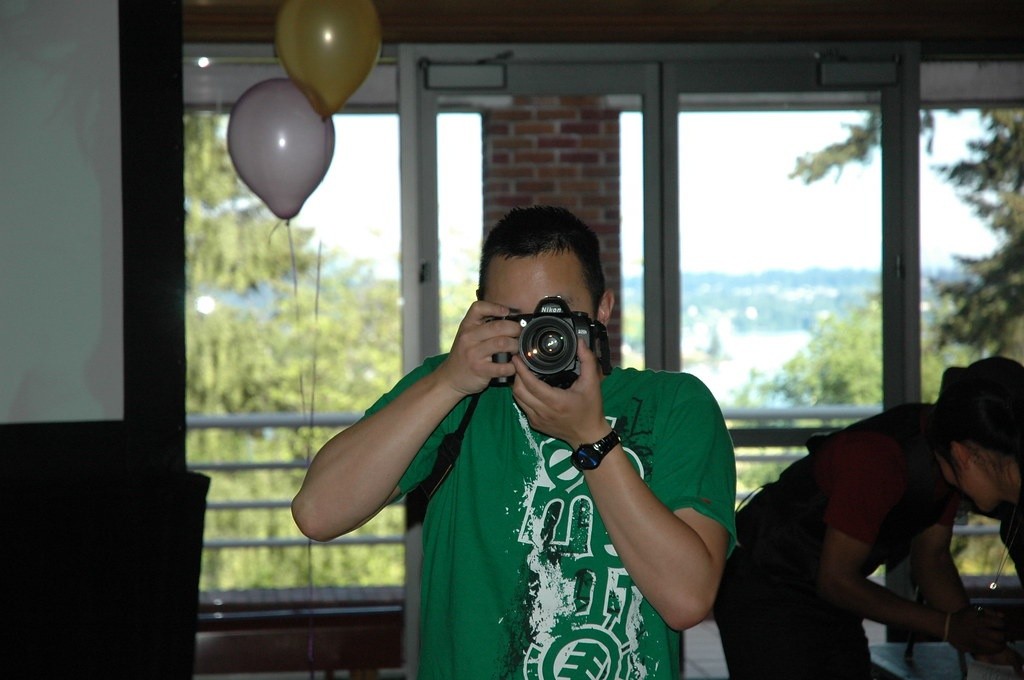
571;428;621;471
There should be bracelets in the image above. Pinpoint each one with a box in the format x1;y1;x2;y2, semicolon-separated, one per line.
941;612;953;642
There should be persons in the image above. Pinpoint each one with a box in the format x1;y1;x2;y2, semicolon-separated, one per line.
291;203;741;680
714;356;1024;680
921;357;1023;593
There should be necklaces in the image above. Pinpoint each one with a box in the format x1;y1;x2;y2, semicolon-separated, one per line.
987;501;1024;591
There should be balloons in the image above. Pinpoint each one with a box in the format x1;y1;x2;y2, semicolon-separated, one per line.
226;78;338;224
271;0;381;124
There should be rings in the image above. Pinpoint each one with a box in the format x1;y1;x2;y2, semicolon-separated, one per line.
974;603;986;617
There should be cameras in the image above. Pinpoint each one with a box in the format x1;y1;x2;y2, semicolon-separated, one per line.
486;296;599;388
